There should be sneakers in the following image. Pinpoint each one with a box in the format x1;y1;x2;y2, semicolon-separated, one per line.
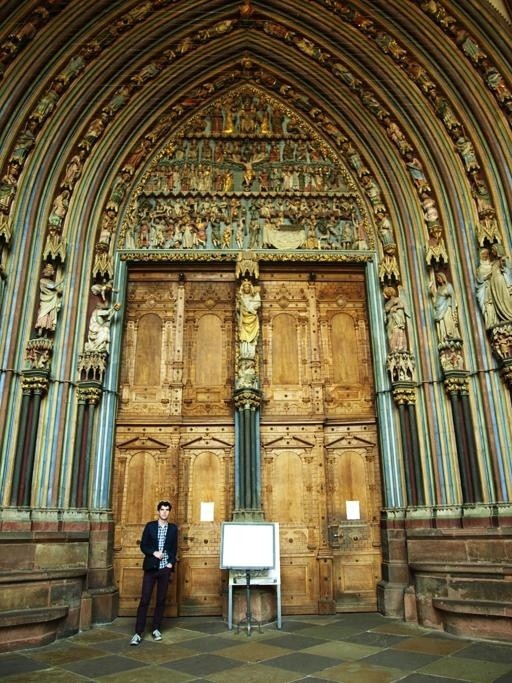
152;629;162;641
129;631;145;645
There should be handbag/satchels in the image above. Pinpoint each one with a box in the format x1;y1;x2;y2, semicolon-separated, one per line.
142;556;160;571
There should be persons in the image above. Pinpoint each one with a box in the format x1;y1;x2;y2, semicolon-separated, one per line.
2;0;511;387
128;500;177;647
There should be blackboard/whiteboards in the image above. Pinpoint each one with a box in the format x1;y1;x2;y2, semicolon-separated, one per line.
219;522;275;570
